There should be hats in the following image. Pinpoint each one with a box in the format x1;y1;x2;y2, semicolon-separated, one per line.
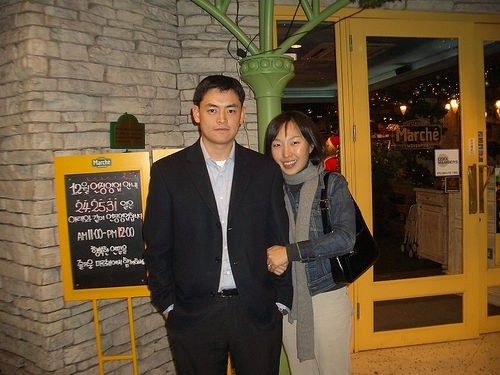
329;136;340;152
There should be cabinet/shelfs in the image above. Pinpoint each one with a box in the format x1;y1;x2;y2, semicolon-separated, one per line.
410;185;500;308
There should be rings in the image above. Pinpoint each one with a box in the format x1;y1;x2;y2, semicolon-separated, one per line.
267;264;271;267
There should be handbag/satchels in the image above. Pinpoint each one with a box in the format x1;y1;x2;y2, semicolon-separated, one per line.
321;172;380;286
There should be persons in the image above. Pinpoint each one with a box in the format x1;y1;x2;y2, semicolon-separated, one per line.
266;111;356;375
141;75;294;375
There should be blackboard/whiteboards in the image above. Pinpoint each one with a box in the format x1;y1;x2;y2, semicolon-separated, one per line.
54;152;150;303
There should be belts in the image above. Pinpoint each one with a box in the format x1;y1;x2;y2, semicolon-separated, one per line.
217;289;239;298
